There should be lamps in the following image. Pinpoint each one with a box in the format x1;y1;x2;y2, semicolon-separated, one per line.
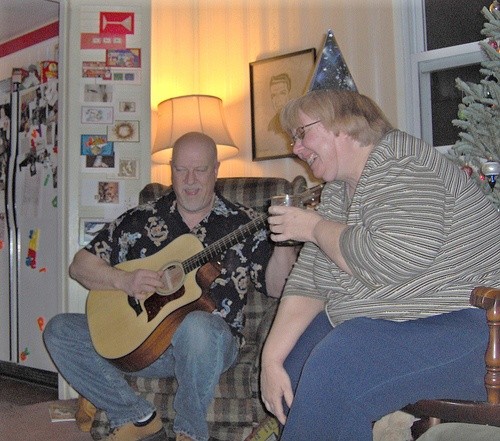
150;96;240;165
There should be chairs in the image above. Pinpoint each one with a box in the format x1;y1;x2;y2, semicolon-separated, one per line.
392;286;500;441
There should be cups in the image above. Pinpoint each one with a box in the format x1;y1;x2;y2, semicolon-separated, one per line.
270;195;306;245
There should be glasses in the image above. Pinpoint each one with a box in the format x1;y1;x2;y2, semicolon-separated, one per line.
289;120;321;147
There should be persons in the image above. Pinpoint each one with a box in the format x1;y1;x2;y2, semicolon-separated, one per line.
42;131;295;441
261;90;500;441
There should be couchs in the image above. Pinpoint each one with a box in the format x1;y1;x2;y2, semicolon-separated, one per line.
75;175;308;441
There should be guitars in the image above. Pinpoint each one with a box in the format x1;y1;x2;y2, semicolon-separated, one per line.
85;181;326;373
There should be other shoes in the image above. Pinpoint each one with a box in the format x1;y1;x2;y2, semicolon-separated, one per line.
104;411;166;441
175;432;195;441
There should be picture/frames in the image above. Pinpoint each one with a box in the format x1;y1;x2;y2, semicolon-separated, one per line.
249;47;316;161
